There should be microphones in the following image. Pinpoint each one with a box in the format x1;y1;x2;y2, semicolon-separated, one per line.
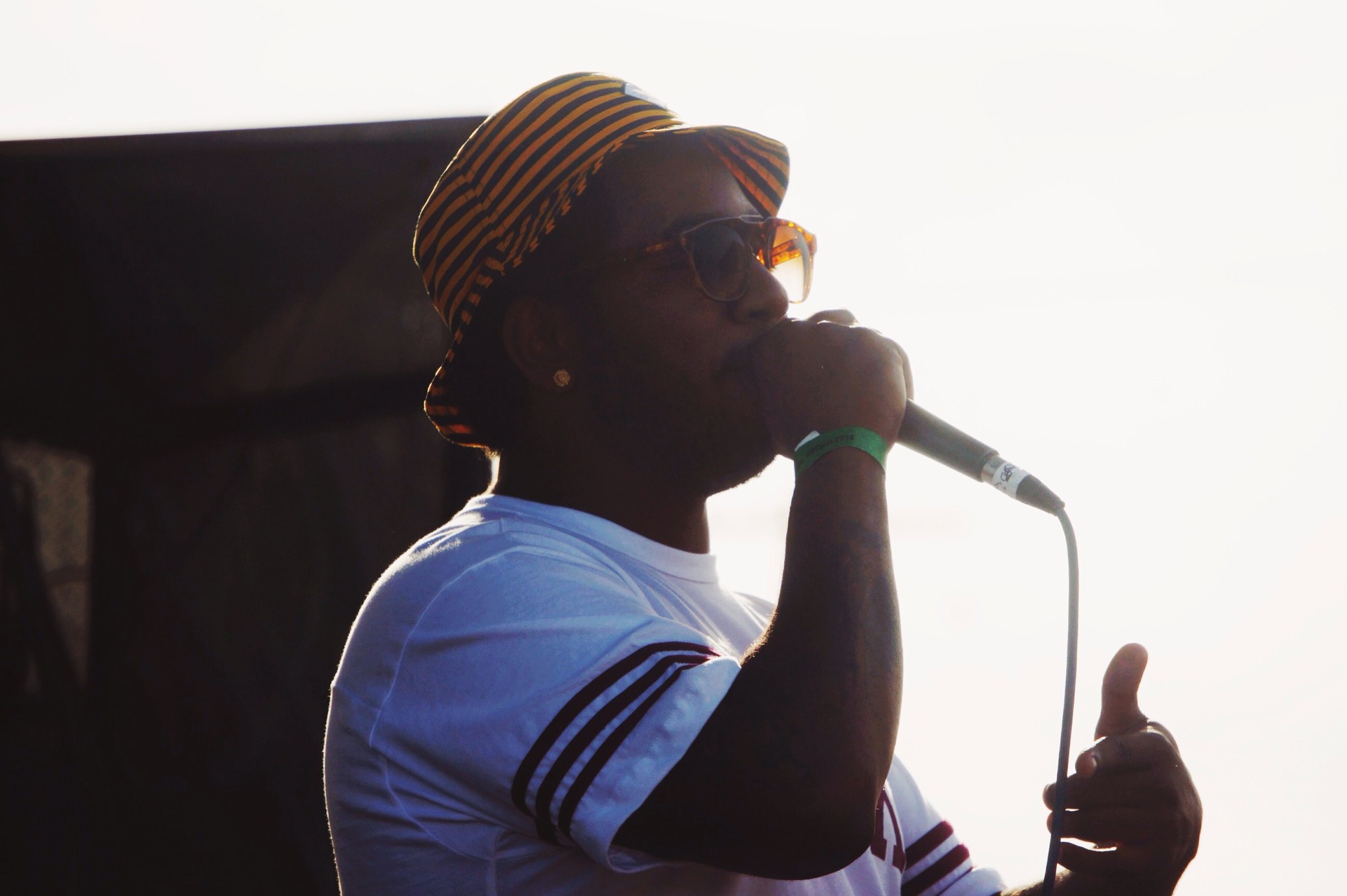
895;399;1065;516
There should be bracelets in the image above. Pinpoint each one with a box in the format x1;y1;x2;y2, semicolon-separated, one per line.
793;424;890;479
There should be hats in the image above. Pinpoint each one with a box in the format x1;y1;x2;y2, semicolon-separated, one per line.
412;72;788;449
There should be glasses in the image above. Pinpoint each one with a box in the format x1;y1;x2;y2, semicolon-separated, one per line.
532;214;817;304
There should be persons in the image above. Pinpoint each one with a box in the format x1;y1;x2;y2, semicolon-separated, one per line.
328;73;1199;896
